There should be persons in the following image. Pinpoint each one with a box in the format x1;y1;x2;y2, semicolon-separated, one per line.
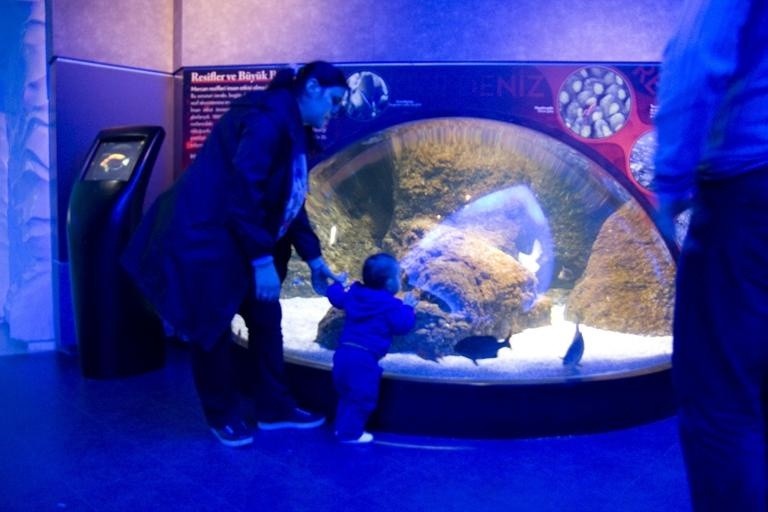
326;252;419;445
646;0;768;512
115;61;351;451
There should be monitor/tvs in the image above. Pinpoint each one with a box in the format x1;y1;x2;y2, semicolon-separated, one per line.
82;137;148;181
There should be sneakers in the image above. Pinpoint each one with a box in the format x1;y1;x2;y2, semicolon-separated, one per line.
258;406;327;431
210;418;253;447
342;431;374;444
